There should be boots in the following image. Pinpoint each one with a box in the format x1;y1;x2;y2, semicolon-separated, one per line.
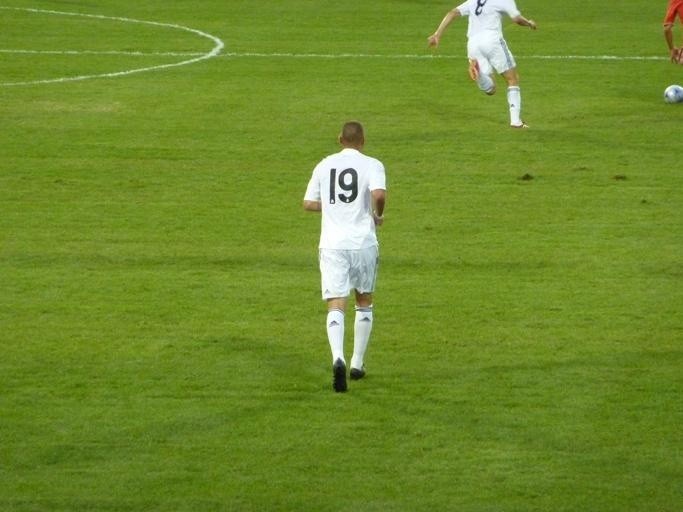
349;367;367;380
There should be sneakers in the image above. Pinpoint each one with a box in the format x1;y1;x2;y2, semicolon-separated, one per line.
332;357;347;393
510;120;528;128
469;56;479;81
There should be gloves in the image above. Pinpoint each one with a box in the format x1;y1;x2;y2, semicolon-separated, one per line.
373;210;384;225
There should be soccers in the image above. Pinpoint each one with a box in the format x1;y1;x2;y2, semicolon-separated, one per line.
664;85;683;104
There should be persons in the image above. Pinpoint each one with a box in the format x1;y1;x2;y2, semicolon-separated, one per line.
428;0;537;128
664;0;683;65
304;122;386;392
